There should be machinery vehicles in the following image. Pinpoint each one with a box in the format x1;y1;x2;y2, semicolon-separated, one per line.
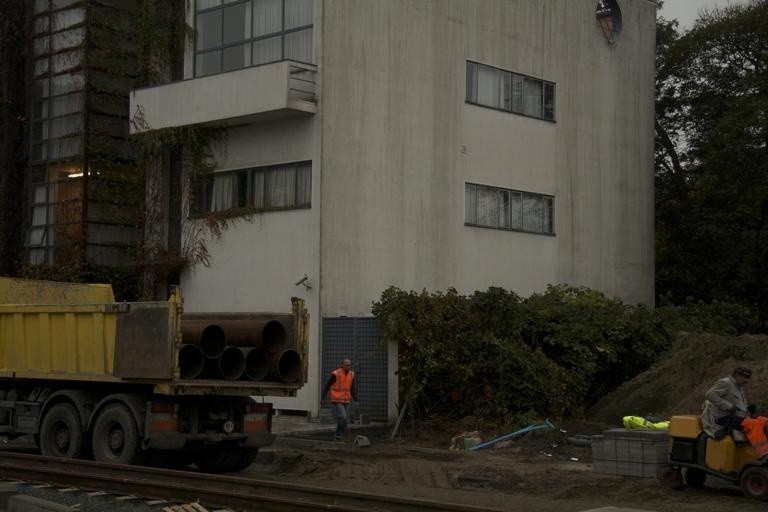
665;414;768;501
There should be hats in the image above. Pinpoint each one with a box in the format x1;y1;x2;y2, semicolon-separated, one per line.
734;366;752;378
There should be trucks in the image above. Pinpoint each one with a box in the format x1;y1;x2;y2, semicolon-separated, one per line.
0;275;312;474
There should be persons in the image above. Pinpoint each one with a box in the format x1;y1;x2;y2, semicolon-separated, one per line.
700;365;754;449
321;358;362;441
